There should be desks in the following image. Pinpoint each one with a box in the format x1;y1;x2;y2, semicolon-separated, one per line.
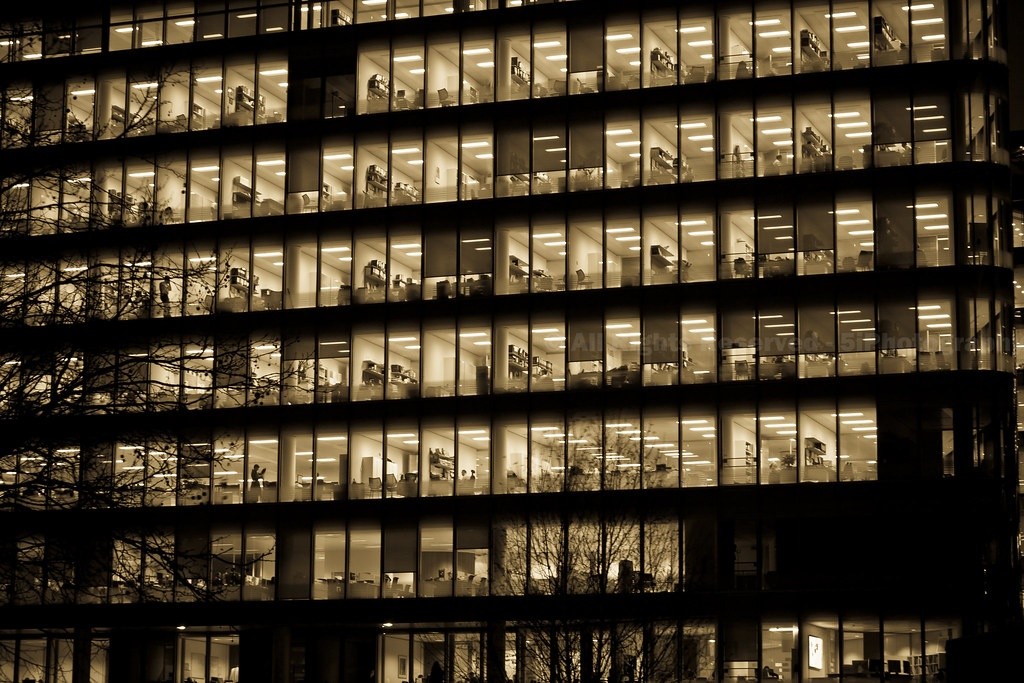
422;580;472;598
805;366;829;378
350;583;380;598
881;357;913;374
213;484;241;503
302;483;333;500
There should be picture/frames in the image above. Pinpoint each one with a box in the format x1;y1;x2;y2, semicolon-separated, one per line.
398;655;407;679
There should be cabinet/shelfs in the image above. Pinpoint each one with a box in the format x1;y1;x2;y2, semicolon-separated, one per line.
365;264;385;283
367;171;387;192
802;133;823;157
652;147;674;169
368;79;389;98
231;267;260;295
112;105;138;124
800;32;822;63
463;85;480;104
509;255;528;277
331;9;352;26
651;245;675;268
233;176;262;204
235;86;265;117
173;343;947;683
322;181;335;204
511;65;528;86
651;51;674;76
874;16;896;49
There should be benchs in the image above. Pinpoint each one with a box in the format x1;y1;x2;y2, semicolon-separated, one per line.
840;460;870;481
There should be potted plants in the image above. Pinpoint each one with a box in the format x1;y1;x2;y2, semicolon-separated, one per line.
779;453;797;483
767;463;778;483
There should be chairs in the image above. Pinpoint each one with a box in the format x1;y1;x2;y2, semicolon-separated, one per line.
200;295;213;315
555;274;565;291
900;146;921;164
395;90;410;111
935;351;951;370
856;250;874;271
302;194;318;213
369;477;382;499
733;257;751;277
684;66;705;83
734;360;750;380
386;474;397;497
437;88;455;106
265;290;282;310
576;269;594;290
403;584;411;598
549;80;566;96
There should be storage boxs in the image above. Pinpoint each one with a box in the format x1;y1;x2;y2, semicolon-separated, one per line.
396;182;418;196
814;443;826;453
801;33;812;38
231;268;247;286
